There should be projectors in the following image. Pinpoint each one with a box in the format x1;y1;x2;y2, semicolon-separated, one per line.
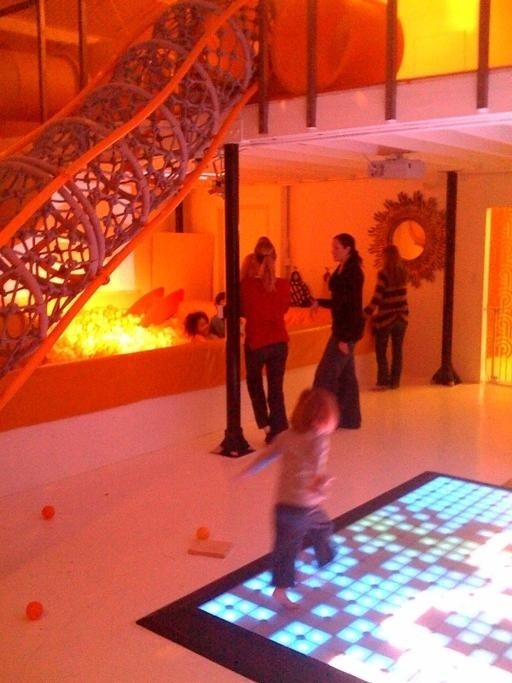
368;158;426;179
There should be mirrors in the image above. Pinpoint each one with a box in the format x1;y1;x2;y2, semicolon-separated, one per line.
368;191;448;289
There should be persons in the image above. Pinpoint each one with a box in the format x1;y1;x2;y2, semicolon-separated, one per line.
313;234;366;429
235;387;340;610
184;311;218;342
240;237;291;444
363;245;410;391
210;292;226;339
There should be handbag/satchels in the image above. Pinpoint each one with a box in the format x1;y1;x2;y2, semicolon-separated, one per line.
288;270;314;307
